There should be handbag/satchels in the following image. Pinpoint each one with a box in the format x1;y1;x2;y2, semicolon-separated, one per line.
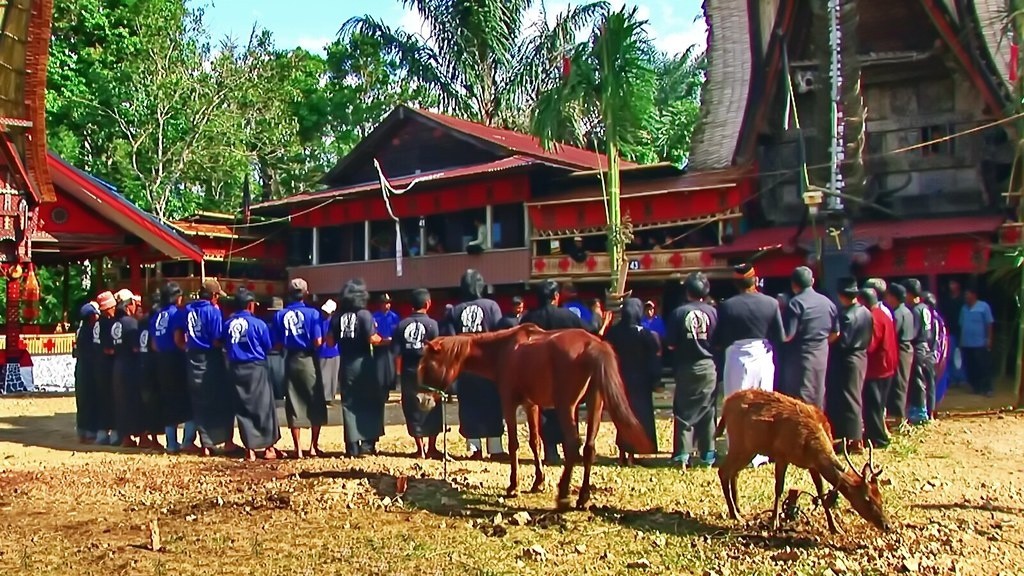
954;346;963;369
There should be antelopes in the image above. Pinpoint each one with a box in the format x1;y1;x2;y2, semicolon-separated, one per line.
712;390;894;536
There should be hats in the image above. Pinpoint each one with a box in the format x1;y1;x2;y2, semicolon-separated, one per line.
835;275;858;293
379;293;392;302
202;280;227;296
267;296;284;311
80;303;101;317
321;299;337;314
645;300;655;307
114;288;141;302
97;291;117;310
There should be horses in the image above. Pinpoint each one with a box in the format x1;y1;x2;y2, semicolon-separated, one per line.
416;322;653;510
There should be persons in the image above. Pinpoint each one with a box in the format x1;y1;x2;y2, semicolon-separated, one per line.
69;212;996;461
